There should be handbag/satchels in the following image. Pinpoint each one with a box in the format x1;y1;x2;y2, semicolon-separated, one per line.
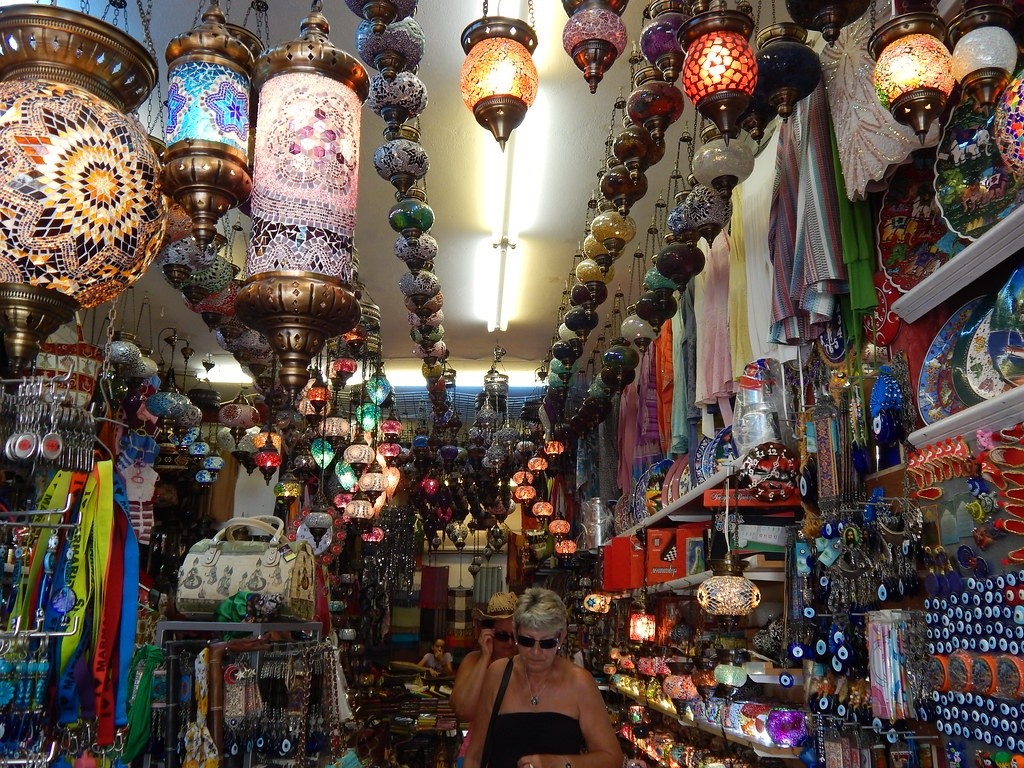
175;515;316;622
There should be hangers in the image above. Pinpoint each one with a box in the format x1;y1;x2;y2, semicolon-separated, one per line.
139;384;148;396
136;401;149;421
133;448;160;481
119;417;148;447
130;467;144;483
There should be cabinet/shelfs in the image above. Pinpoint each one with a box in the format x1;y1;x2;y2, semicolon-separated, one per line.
889;202;1024;450
599;453;805;767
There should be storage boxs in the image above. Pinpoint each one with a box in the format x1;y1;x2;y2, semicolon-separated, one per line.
603;544;622;591
646;527;676;586
629;535;645;589
611;536;630;590
676;529;704;579
703;490;802;508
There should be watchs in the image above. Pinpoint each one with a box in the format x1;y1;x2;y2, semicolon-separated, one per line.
564;757;572;768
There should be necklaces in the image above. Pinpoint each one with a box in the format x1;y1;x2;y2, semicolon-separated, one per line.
525;667;548;705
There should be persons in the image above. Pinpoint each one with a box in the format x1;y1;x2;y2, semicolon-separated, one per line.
463;588;624;768
449;592;519;768
417;637;453;678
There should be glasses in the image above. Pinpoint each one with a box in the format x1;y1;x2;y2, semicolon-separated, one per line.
493;630;516;641
516;634;561;649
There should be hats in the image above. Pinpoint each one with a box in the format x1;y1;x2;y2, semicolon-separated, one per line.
471;592;519;621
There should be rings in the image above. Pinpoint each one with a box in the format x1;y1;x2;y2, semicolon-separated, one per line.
530;764;533;768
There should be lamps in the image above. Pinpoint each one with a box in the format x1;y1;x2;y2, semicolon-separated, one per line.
0;0;1024;768
